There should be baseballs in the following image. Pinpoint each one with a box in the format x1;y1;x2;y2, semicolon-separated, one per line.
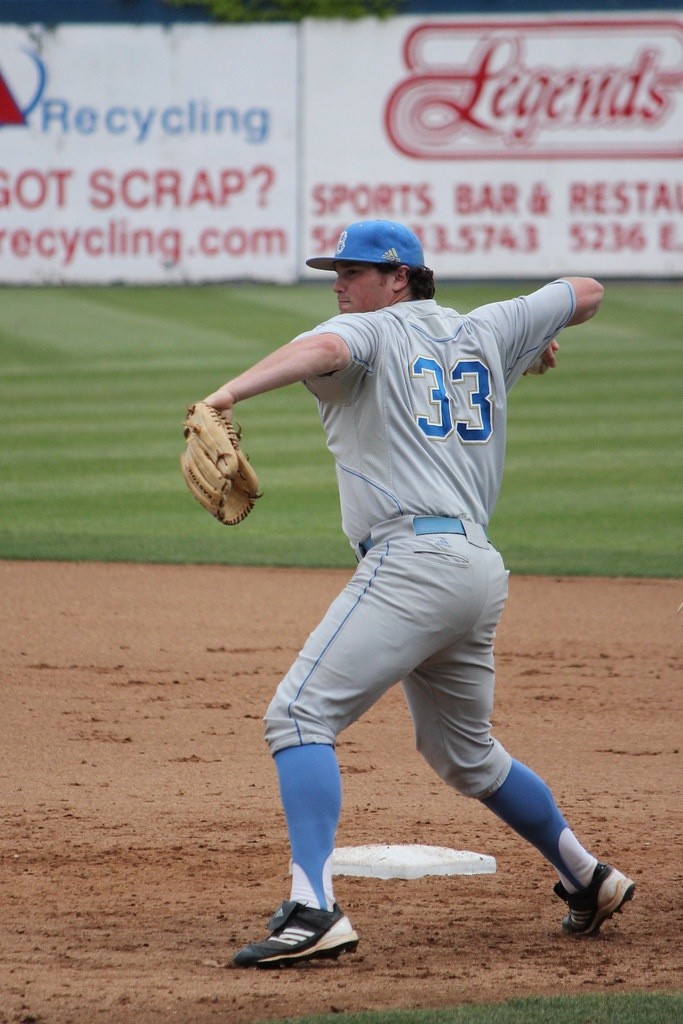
528;355;549;375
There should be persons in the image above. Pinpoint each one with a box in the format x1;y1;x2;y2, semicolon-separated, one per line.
199;220;637;970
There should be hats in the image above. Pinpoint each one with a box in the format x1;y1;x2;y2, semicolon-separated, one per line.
305;218;424;270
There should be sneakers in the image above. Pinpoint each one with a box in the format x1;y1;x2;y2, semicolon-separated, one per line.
553;861;637;935
233;901;359;968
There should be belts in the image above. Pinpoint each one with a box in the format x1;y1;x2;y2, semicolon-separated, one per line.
359;518;492;556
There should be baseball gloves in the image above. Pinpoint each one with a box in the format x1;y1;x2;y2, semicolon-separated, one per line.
179;400;265;526
